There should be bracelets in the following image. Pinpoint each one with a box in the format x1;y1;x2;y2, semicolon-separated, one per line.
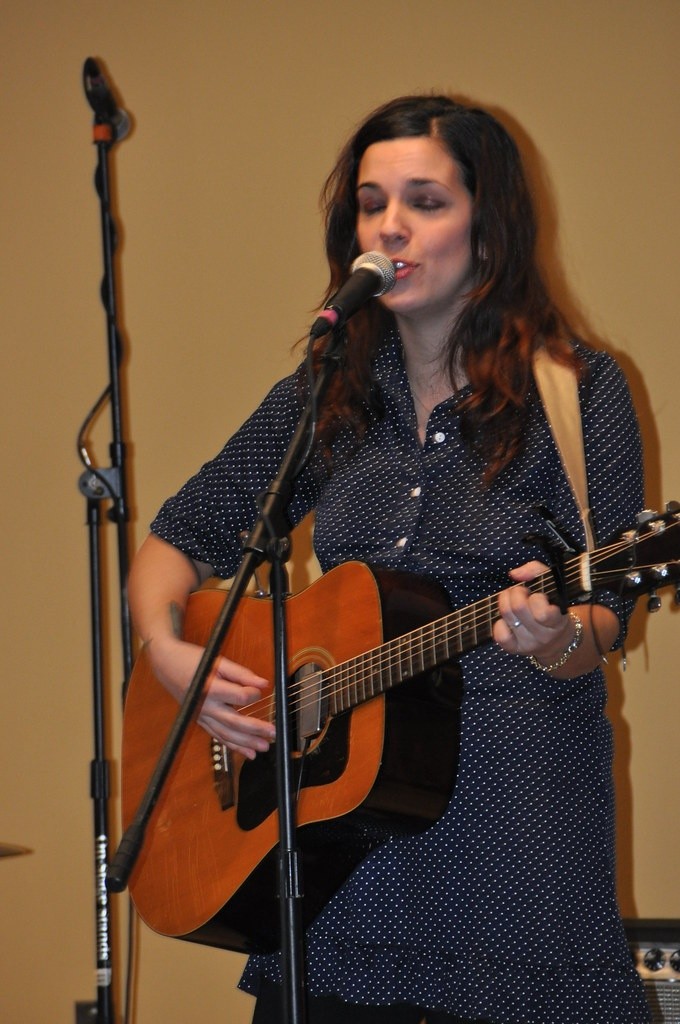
529;614;585;671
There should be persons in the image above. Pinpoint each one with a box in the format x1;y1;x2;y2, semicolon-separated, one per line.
124;93;657;1023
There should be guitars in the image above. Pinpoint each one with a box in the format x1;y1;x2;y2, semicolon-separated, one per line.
119;502;680;956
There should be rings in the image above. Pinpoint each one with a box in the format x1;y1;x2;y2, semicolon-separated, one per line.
510;621;522;630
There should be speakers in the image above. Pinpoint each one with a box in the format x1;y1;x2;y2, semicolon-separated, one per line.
623;918;680;1024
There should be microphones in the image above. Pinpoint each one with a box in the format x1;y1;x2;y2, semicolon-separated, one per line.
87;58;130;142
311;250;396;339
